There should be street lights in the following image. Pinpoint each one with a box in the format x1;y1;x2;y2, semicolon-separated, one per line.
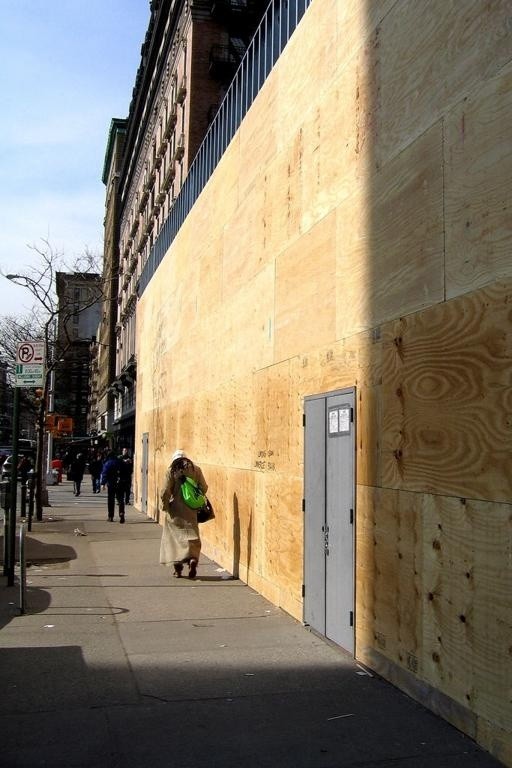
6;274;58;486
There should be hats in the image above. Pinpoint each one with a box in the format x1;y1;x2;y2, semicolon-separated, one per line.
172;450;186;461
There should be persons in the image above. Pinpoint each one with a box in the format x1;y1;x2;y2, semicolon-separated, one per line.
17;454;33;482
160;449;209;579
57;444;133;524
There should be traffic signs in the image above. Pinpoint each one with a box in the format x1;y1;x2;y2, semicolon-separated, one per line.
15;364;44;387
16;341;45;364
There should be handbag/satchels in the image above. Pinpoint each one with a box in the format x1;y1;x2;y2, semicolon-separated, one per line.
197;499;212;522
179;475;207;509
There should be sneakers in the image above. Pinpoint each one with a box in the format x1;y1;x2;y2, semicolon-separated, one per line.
173;571;181;578
120;512;124;523
189;559;197;578
107;517;113;522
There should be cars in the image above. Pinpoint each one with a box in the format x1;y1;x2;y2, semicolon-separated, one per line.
1;454;34;480
